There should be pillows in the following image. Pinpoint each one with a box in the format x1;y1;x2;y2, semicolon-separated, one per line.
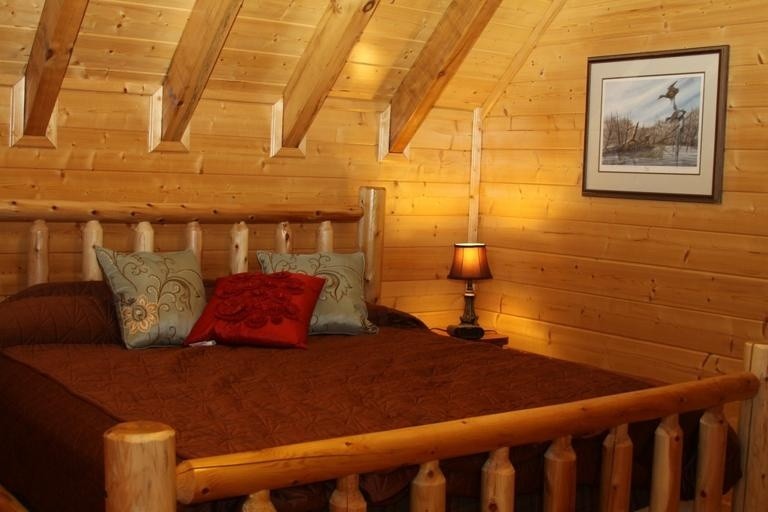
94;245;382;350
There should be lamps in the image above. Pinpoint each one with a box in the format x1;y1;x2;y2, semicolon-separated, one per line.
446;242;494;324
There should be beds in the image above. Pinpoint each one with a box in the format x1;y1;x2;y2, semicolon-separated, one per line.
1;185;768;512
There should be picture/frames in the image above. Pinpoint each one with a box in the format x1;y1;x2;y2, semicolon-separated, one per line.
583;45;730;202
